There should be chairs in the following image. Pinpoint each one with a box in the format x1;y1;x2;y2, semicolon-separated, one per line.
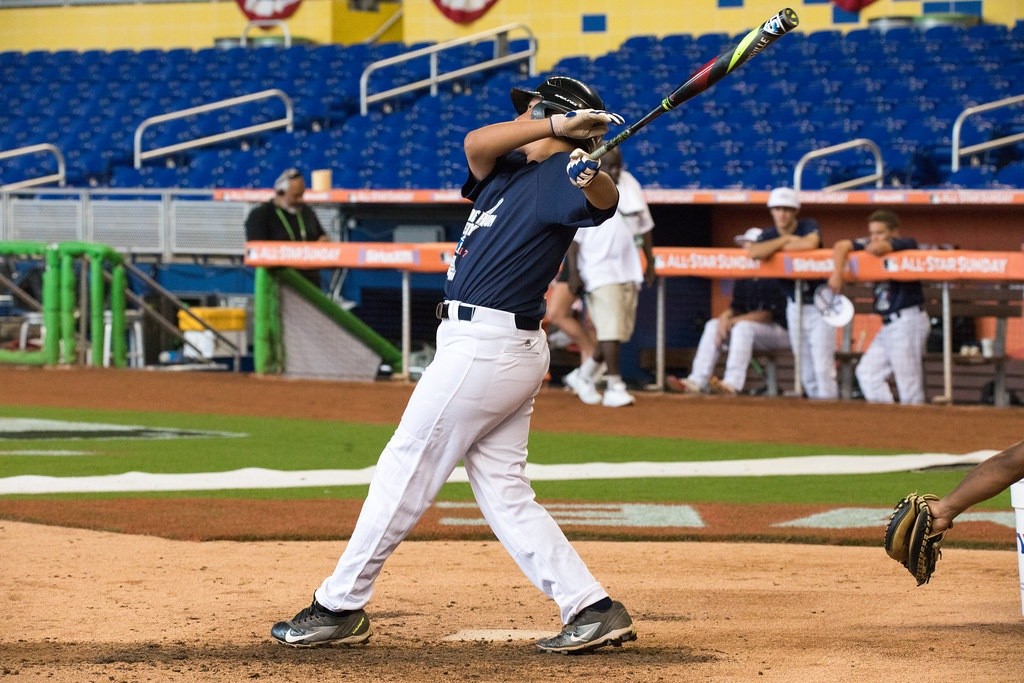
0;21;1024;399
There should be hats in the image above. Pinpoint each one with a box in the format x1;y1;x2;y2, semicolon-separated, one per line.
814;285;855;328
767;187;800;210
733;227;762;243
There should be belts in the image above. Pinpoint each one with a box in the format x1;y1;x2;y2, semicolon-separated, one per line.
882;305;923;324
436;302;540;331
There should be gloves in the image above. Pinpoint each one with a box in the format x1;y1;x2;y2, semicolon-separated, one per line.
550;108;625;140
566;148;601;189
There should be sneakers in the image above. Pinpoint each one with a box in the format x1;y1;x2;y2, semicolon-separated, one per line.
709;376;743;397
603;382;635;407
563;368;602;404
535;601;638;655
271;590;372;647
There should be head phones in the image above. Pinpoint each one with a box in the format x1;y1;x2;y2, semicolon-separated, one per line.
274;170;301;196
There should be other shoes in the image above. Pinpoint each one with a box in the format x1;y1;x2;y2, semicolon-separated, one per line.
666;375;702;394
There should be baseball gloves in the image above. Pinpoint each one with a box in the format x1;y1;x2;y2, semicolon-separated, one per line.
883;494;953;582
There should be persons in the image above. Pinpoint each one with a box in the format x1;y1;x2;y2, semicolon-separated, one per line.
243;166;334;289
747;186;840;401
272;73;637;657
543;140;655;409
665;226;791;397
882;442;1024;588
827;211;932;409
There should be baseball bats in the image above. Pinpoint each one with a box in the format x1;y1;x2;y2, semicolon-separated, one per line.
588;7;800;163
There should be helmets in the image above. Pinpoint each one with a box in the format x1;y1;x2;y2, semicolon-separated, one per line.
510;76;607;153
